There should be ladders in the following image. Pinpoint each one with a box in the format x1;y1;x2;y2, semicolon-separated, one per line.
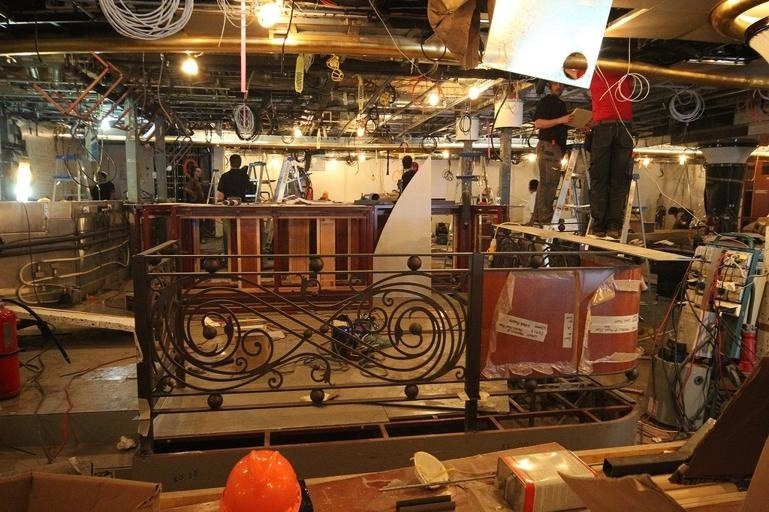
543;143;592;252
51;154;92;202
582;173;656;328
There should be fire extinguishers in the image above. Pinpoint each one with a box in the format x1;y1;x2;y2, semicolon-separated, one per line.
264;156;304;254
245;161;274;203
0;298;71;401
206;169;222;204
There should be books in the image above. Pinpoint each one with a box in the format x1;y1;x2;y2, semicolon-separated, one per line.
564;107;592;130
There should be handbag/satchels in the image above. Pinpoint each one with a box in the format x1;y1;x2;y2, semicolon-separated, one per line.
584;131;594;151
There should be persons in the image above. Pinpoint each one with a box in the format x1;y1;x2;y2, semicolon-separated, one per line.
655;193;666;228
92;172;117;203
184;166;206;202
522;179;539;227
668;207;694;224
532;81;591;225
568;68;634;236
401;155;420;194
216;154;250;203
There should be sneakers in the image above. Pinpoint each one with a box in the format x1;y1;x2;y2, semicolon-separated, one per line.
594;230;619;238
